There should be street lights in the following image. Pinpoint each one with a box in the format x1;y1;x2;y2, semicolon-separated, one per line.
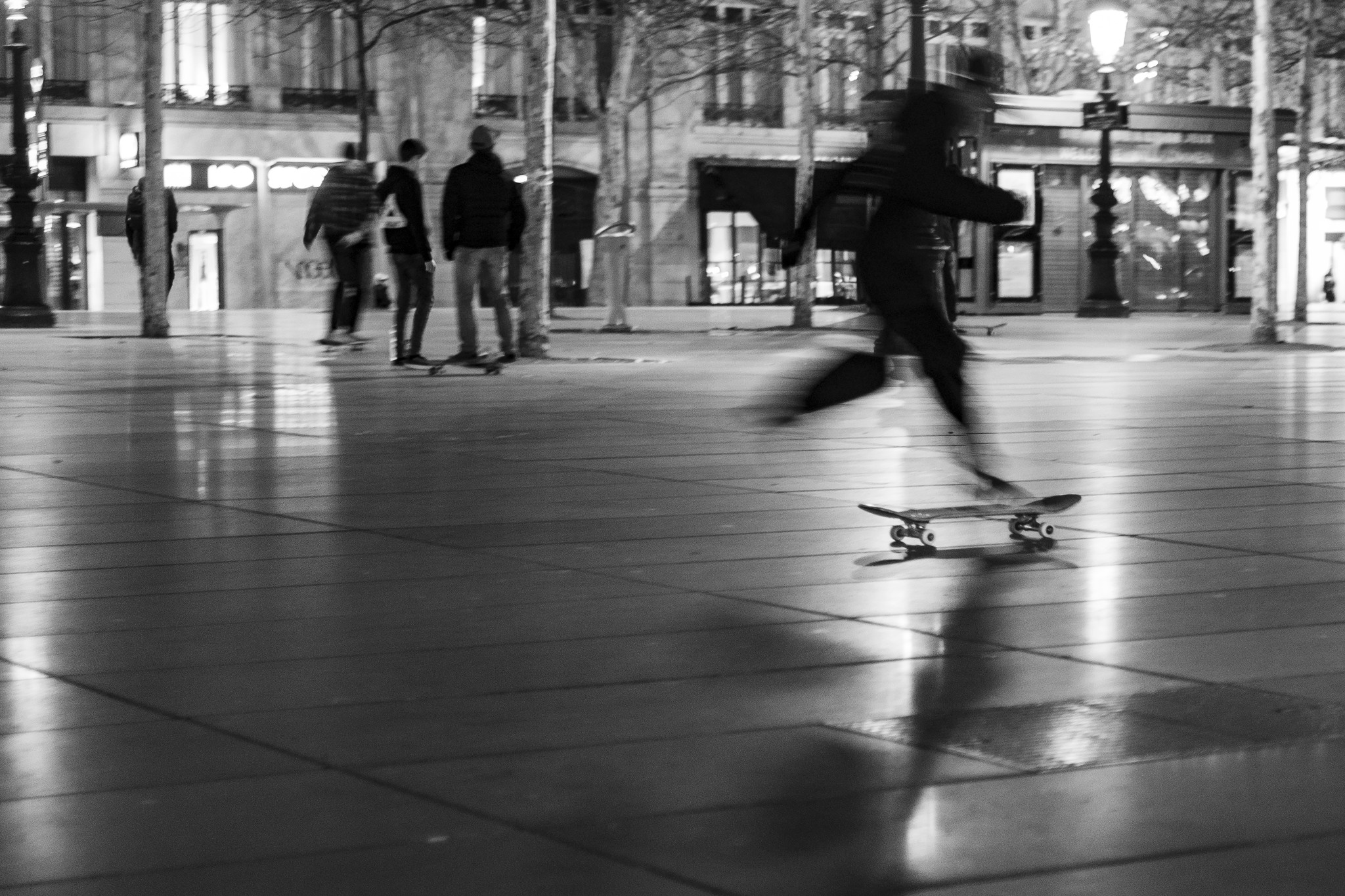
0;0;57;330
1077;11;1131;319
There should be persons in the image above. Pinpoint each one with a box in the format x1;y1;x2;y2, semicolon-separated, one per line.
441;126;527;363
753;85;1026;499
126;176;179;300
303;142;379;345
374;138;436;366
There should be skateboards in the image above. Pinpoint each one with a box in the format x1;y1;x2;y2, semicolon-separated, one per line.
858;495;1083;544
317;338;375;353
445;359;507;375
953;322;1006;337
398;360;443;375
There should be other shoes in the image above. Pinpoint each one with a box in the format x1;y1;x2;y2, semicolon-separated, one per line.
498;353;516;363
450;350;478;361
390;355;403;366
405;353;429;366
